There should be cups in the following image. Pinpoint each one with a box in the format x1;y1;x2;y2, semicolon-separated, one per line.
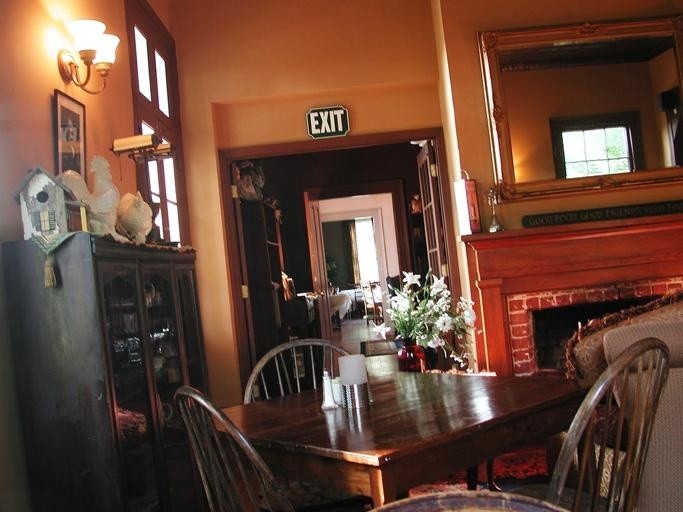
337;353;368;386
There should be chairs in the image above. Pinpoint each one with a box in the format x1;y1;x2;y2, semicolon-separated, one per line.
564;288;683;511
371;338;671;511
174;384;371;512
243;339;352;405
348;279;384;329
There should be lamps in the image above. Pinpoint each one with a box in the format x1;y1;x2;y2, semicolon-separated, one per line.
56;17;121;97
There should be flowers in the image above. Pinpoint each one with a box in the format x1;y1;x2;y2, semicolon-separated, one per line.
381;268;483;367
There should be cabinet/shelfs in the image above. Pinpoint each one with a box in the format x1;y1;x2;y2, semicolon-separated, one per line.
281;298;323;394
240;199;287;401
0;231;212;512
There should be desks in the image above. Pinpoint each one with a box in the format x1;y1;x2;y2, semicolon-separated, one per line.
329;289;356;330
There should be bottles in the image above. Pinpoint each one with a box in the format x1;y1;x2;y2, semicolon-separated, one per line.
321;367;337;411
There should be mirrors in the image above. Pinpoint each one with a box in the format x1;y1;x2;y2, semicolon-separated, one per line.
475;14;682;204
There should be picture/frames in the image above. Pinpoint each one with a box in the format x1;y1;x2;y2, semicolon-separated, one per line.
54;87;87;187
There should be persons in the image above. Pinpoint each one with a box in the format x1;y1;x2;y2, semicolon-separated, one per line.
62;117;80;174
